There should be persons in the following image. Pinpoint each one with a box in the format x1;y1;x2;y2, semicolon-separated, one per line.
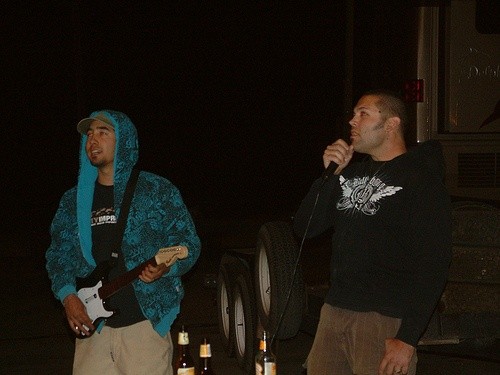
292;89;453;375
44;110;201;375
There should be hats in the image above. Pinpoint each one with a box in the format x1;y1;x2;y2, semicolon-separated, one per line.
77;112;116;135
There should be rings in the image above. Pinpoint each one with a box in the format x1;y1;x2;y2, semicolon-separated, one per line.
395;370;400;373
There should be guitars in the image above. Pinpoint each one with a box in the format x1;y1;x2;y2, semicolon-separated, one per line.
62;245;189;339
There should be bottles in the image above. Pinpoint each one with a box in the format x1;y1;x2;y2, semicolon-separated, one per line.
199;336;215;375
173;325;196;375
254;331;276;375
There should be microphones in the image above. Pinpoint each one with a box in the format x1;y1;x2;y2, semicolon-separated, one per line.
325;138;351;178
